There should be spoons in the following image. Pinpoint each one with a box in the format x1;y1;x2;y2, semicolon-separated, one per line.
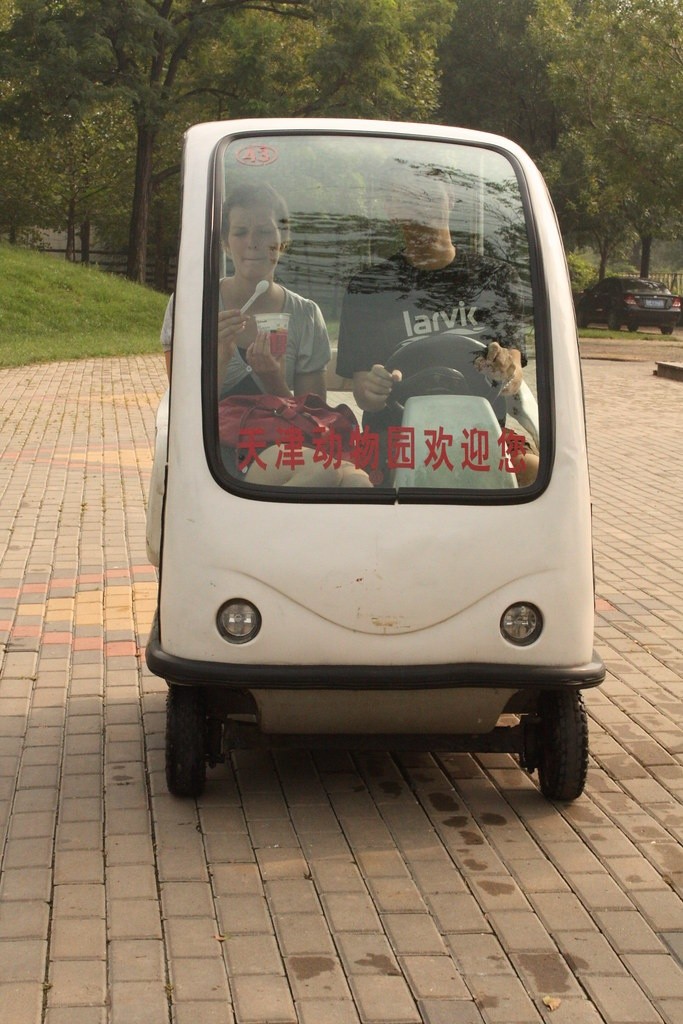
240;280;269;314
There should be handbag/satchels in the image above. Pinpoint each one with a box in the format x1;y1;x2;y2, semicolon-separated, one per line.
215;391;360;452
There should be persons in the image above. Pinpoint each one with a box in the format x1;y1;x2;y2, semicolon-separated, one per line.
335;159;540;488
160;178;332;483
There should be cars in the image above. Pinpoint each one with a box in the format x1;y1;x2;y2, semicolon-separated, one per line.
572;275;682;336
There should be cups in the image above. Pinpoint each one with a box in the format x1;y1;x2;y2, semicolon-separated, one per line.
254;313;292;354
